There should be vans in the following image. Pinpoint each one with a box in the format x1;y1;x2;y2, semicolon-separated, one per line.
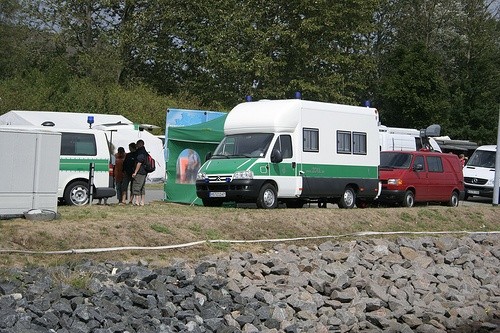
1;124;117;206
195;99;381;210
356;151;465;208
462;145;499;202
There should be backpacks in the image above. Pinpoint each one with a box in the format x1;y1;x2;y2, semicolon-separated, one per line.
146;151;155;173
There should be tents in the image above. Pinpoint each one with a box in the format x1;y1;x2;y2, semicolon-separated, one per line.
0;110;229;206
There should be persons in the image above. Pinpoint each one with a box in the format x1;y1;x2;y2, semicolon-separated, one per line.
113;140;150;206
186;150;199;183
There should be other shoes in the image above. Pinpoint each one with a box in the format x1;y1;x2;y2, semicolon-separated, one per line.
129;201;133;203
119;202;126;206
140;201;144;205
134;201;140;206
